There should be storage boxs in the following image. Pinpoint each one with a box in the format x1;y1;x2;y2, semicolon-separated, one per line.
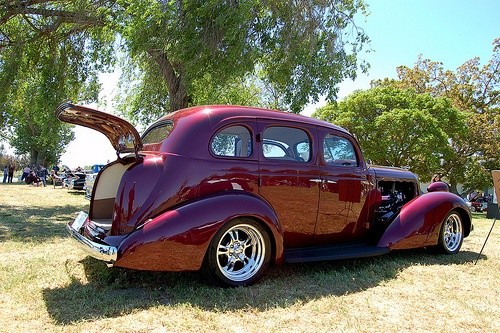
486;202;500;220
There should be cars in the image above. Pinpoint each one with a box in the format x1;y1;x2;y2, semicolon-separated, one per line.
54;102;474;290
56;164;107;193
466;197;489;212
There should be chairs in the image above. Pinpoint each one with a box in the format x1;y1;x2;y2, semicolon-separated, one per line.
33;171;63;188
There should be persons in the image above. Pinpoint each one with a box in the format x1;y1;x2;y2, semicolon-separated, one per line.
468;190;492;202
2;166;8;183
7;163;15;184
21;163;83;185
427;174;450;192
